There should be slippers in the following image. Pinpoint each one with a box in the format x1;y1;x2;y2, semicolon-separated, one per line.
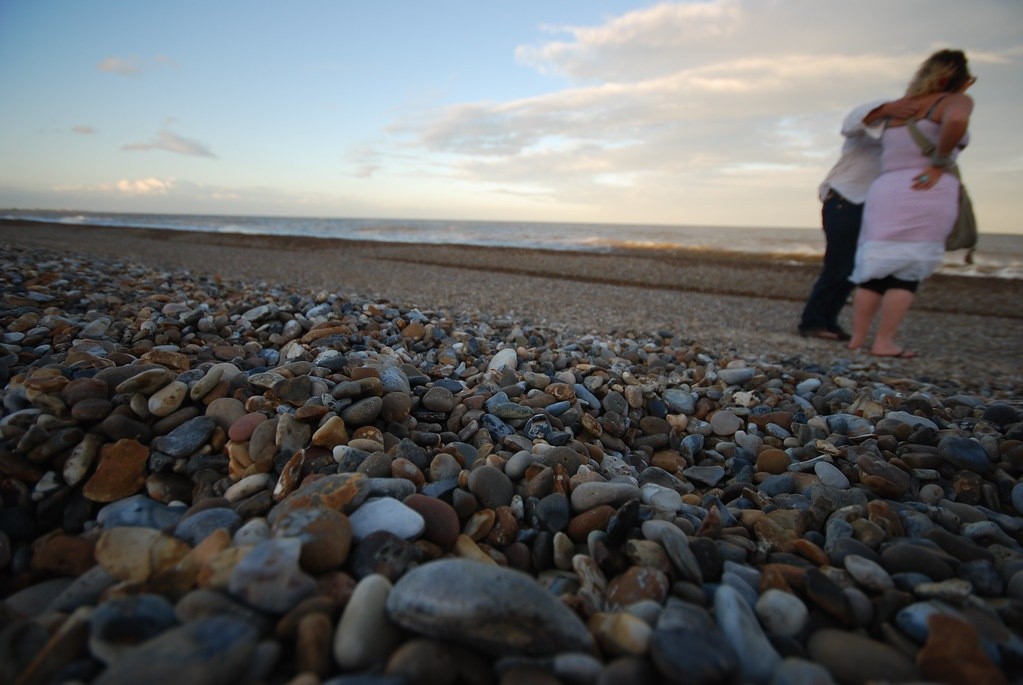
872;343;921;358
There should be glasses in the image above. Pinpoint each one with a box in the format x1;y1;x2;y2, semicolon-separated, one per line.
960;75;977;88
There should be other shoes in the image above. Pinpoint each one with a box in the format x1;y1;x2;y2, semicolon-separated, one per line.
798;322;851;340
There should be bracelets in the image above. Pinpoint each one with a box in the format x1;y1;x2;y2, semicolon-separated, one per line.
928;150;950;167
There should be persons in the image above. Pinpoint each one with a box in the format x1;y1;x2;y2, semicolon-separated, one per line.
798;94;928;341
847;48;976;359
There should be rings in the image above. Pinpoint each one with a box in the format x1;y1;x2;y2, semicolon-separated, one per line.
920;176;929;182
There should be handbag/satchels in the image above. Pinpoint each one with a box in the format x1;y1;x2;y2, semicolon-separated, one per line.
946;187;978;267
